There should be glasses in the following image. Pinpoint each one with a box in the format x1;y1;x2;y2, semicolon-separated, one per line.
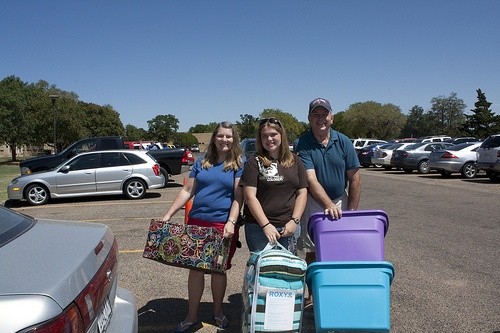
259;118;281;128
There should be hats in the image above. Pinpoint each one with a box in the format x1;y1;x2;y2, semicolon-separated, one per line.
309;98;332;116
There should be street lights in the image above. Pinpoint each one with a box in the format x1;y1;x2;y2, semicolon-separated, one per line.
49;95;59;155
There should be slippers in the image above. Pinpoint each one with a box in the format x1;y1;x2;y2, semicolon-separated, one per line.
177;319;196;332
215;316;227;328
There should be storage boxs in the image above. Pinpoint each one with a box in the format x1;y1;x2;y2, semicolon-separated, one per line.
307;209;390;262
307;258;395;333
142;219;231;275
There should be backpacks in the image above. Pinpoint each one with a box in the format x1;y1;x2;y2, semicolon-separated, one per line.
241;239;307;333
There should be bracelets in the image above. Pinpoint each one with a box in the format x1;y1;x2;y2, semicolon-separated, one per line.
261;222;270;229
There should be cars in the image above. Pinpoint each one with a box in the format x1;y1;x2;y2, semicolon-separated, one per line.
350;134;500;182
0;203;138;333
7;149;165;206
239;138;256;159
124;141;200;166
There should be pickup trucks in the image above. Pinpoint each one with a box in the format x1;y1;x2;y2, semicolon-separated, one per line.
19;136;185;188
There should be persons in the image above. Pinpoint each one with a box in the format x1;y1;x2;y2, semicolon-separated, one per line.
155;121;246;333
294;98;362;317
239;117;308;253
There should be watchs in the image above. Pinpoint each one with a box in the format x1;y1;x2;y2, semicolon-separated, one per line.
228;219;236;225
291;217;300;224
